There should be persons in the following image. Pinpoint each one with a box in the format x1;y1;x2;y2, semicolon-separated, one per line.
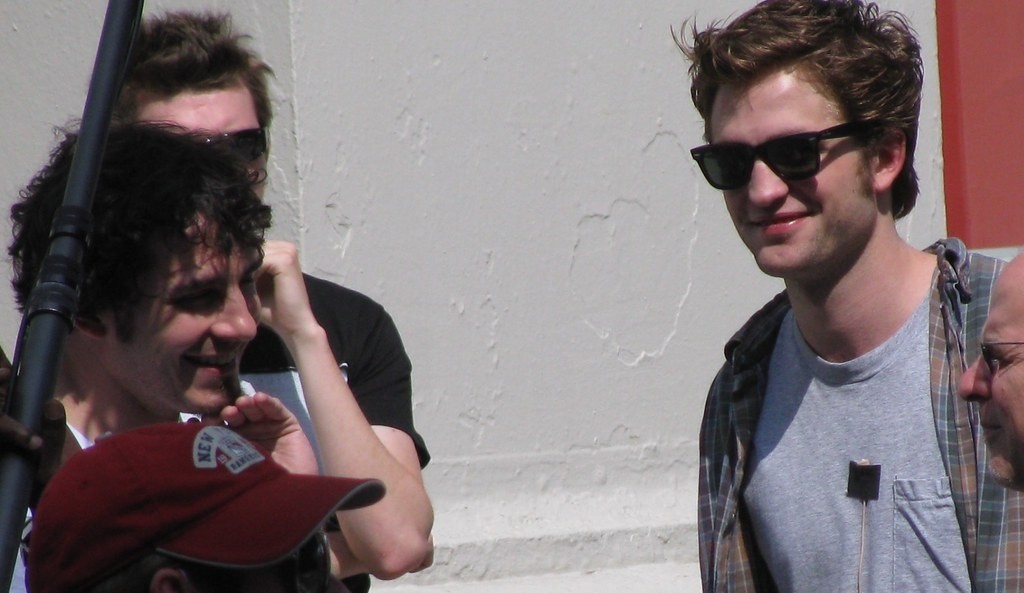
106;10;434;593
6;125;323;593
956;249;1024;490
670;2;1023;593
25;423;385;593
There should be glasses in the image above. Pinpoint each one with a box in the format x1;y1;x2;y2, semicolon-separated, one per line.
979;342;1024;377
689;117;885;191
208;128;269;161
207;532;330;593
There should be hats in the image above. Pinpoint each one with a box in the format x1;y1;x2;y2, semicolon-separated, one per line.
25;422;387;593
